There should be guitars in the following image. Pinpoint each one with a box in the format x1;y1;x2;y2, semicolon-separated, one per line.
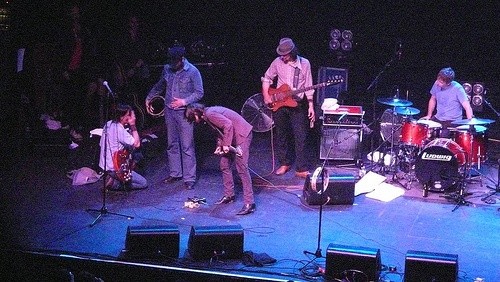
113;138;143;183
216;136;244;159
267;75;344;112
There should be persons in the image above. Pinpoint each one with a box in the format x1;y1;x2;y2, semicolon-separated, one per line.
66;4;149;107
261;38;315;179
145;47;204;189
99;105;148;189
183;103;257;216
418;67;473;137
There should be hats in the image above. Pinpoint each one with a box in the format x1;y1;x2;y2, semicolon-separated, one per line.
438;67;454;79
166;46;185;63
277;38;295;56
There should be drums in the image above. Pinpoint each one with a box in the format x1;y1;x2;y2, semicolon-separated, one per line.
417;119;443;140
414;137;466;191
453;124;489;164
380;109;413;145
147;95;166;118
400;122;430;148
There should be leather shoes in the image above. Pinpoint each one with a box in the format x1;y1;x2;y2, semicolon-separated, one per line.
184;181;195;189
236;203;256;215
162;176;182;184
213;195;236;205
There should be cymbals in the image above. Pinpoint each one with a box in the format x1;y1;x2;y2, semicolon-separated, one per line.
393;106;420;115
376;97;413;107
451;117;496;125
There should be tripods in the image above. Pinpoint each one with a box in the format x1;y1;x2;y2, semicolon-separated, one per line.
447;130;500;212
366;52;416;189
298;121;342;270
84;92;134;229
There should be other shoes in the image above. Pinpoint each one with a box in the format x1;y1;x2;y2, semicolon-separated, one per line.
97;174;113;191
275;164;291;175
294;170;310;178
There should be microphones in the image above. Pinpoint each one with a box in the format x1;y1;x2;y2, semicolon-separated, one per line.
399;43;401;61
103;80;114;96
338;111;348;121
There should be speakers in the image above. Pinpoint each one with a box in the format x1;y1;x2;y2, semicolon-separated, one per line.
301;172;356;205
188;225;244;261
324;242;381;282
404;250;459;282
125;225;179;260
319;125;361;162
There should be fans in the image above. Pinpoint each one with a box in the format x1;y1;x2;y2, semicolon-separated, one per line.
241;93;276;132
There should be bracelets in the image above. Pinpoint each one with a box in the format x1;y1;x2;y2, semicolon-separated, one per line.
130;124;138;132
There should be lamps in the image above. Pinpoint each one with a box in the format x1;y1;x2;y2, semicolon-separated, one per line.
460;81;485;110
328;29;354;63
